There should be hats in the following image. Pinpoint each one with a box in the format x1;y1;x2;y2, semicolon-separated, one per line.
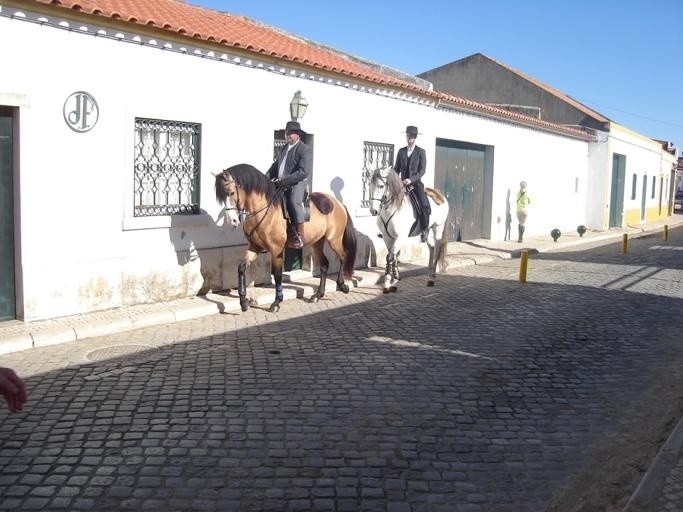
279;122;307;138
399;126;423;136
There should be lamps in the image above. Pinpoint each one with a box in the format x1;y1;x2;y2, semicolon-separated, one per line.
290;89;309;120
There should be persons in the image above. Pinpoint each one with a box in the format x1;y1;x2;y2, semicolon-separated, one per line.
393;124;432;243
516;180;532;242
0;366;28;415
263;119;309;251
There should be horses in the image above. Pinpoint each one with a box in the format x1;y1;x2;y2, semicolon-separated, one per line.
209;163;358;314
366;161;450;295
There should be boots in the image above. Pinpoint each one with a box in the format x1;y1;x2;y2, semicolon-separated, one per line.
421;215;429;242
287;222;304;249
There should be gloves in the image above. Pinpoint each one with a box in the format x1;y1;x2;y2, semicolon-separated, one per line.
270;176;282;188
